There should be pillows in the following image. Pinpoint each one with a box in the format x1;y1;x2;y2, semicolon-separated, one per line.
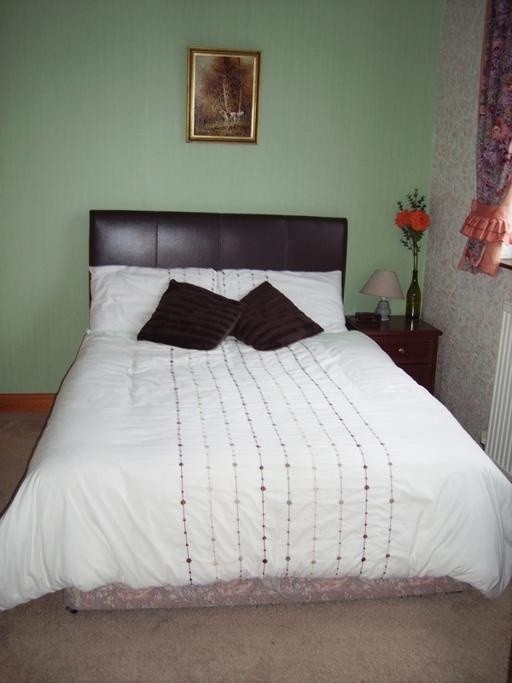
88;265;348;351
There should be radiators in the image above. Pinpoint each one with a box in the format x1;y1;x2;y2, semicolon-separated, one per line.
484;301;512;483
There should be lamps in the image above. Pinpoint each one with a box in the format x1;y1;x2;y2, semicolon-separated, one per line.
359;270;405;321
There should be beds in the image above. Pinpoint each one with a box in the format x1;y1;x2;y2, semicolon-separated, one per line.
0;208;512;615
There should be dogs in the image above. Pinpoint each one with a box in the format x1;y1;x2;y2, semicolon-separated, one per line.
218;109;245;126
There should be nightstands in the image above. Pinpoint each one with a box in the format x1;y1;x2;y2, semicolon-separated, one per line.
346;316;443;405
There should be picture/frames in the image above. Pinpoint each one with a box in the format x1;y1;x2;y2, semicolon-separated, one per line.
185;46;261;145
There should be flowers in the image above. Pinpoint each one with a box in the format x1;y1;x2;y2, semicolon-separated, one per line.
395;189;430;269
